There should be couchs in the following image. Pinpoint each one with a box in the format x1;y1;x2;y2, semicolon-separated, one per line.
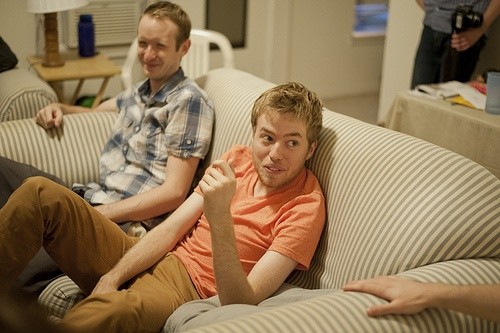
0;66;500;333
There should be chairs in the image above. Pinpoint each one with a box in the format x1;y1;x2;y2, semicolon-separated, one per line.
122;29;236;90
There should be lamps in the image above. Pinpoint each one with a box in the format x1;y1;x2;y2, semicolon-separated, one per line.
25;0;89;67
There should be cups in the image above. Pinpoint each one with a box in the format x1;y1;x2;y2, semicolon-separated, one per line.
485;71;500;115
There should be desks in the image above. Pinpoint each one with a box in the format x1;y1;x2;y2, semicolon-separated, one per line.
25;51;122;108
377;79;500;181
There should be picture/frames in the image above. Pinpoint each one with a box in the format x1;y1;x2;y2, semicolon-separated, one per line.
205;0;247;50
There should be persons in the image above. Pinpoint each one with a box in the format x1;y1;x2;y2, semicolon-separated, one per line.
0;0;215;299
0;83;327;333
342;275;500;323
411;0;500;90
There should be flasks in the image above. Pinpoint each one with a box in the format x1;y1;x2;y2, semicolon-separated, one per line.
77;14;95;58
